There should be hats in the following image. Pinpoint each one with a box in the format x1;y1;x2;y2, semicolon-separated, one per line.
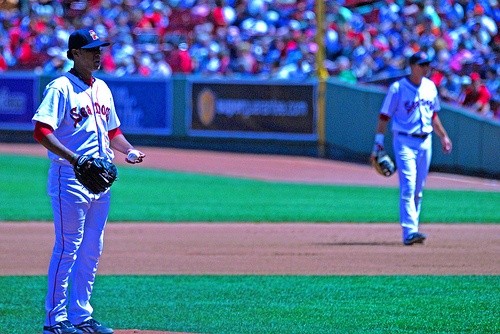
68;29;111;50
409;51;432;64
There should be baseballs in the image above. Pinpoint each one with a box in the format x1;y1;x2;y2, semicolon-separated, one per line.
127;150;140;162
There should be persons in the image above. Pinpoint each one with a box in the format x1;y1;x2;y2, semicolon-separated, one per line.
0;0;318;82
31;30;146;334
372;51;452;245
324;0;500;122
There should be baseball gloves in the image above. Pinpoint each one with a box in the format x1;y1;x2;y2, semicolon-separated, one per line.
370;149;395;177
74;157;118;195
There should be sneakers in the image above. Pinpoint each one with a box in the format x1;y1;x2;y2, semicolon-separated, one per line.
43;319;84;334
72;319;114;334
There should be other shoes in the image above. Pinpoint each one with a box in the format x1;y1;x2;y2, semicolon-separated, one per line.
404;233;426;245
